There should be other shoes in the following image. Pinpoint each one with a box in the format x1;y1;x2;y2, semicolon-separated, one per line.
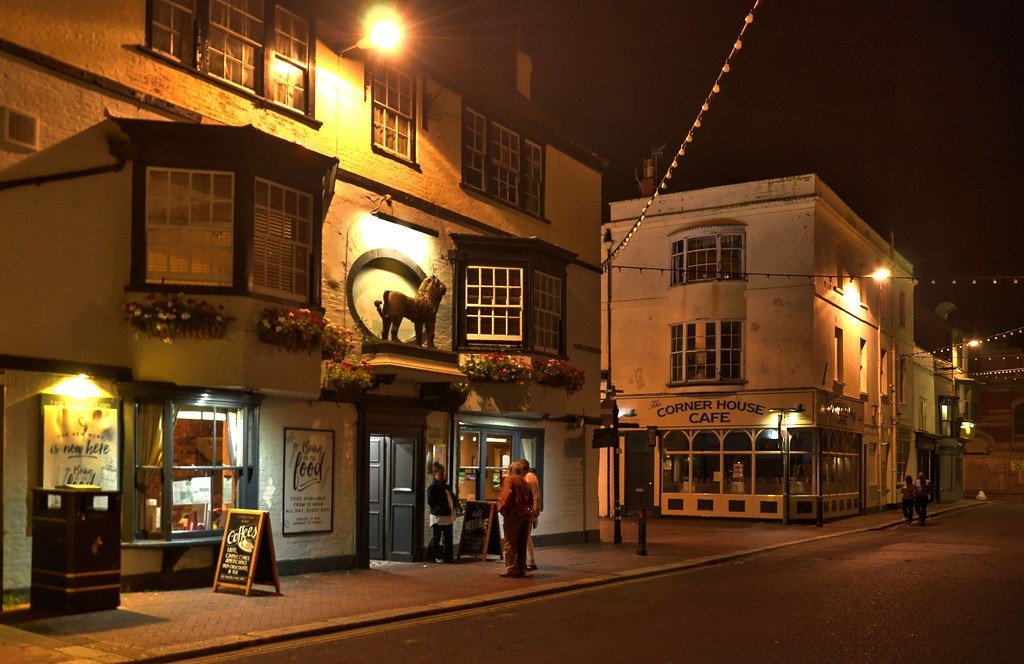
525;565;537;570
908;521;911;524
905;517;908;521
432;557;445;564
917;521;924;526
446;557;457;563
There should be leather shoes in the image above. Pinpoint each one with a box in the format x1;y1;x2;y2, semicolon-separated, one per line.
499;569;526;578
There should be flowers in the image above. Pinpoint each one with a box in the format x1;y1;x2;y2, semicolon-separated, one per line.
319;316;361;359
322;358;374;403
454;352;531;384
120;292;233;344
253;301;331;355
534;358;586;399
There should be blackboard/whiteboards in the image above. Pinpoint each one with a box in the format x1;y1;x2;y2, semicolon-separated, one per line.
459;501;496;557
216;508;266;591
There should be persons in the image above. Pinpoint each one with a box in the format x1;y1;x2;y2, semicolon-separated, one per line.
425;460;463;563
901;475;916;525
914;471;932;526
517;459;541;571
496;461;534;576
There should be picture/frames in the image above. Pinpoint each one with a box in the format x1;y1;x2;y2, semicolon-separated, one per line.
472;456;488;479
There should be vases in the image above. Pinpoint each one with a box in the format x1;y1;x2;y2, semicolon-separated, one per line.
258;322;319;350
322;349;345;362
468;372;519;384
145;322;223;339
318;386;361;404
535;370;571;388
